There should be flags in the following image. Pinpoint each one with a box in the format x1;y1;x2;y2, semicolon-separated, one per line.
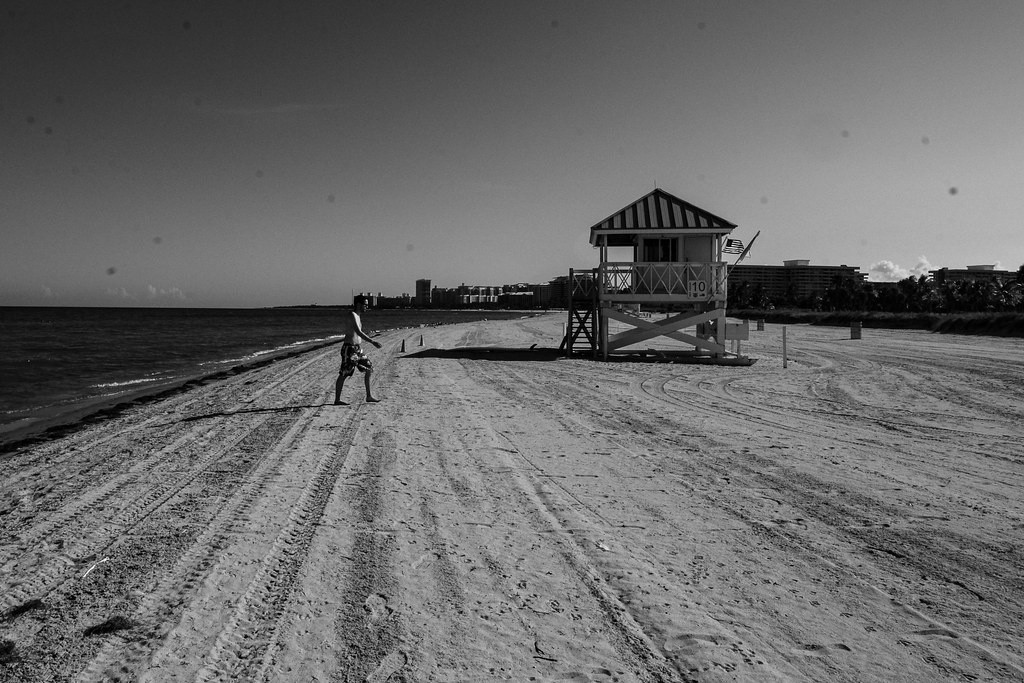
723;239;744;254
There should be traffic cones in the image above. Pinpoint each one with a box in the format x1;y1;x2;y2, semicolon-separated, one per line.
418;335;425;346
399;339;407;353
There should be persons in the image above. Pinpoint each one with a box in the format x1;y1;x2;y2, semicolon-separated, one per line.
334;296;382;405
